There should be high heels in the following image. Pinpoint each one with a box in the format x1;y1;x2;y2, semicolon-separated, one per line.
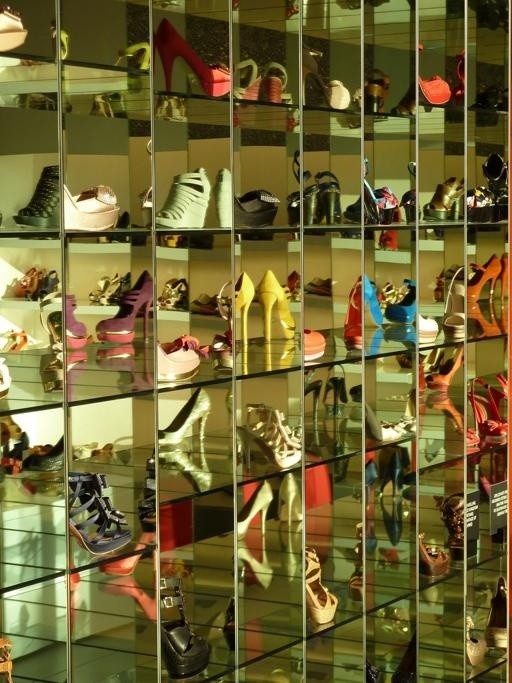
139;139;152;208
65;351;88;400
280;531;297;583
343;276;363;349
213;167;233;227
286;150;300;227
237;404;301;472
304;328;326;362
16;267;36;296
453;49;465;105
158;388;210;445
257;269;296;342
103;577;156;622
304;548;338;624
0;421;10;444
380;451;403;496
261;340;298;371
0;357;10;400
481;151;508;220
263;61;293;104
380;231;397;250
97;345;154;391
9;331;28;352
466;186;496;222
380;280;406;308
234;190;281;226
427;393;464;435
89;275;109;302
39;291;64;392
323;365;348;415
467;303;502;338
156;335;209;383
32;270;60;300
418;314;439;344
364;158;400;224
423;177;464;220
467;255;502;302
304;366;322;419
466;427;481;448
63;184;120;230
96;271;152;344
365;276;383;327
303;170;320;225
233;58;262;101
302;42;351;110
400;388;416;433
496;253;507;299
387;279;417;323
319;417;343;455
99;273;120;305
23;436;64;471
364;69;391;113
13;165;59;226
212;280;233;372
489;374;509;411
399;161;415;224
278;473;302;527
422;348;444;373
466;616;486;665
392;632;416;683
158;447;212;493
350;385;362;403
304;169;341;226
349;523;364;600
469;85;498;112
486;576;509;651
392;86;415;115
237;479;275;540
67;471;132;555
0;330;16;352
25;270;45;299
365;461;379;502
366;404;403;441
0;4;29;52
442;493;464;560
343;199;361;223
442;266;465;339
366;660;386;683
305;420;322;458
65;295;93;349
159;577;212;679
426;346;464;392
108;272;131;304
419;531;449;575
155;167;211;228
237;540;272;589
468;377;507;449
100;533;155;576
156;18;231;97
235;271;255;345
157;279;188;310
364;328;383;355
419;43;451;105
498;88;508;110
0;415;22;440
381;496;403;546
385;325;416;347
496;299;508;334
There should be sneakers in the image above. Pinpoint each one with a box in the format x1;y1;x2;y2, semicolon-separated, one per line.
306;277;332;296
189;294;232;314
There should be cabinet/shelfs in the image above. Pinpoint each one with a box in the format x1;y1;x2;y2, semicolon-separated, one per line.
2;0;512;682
